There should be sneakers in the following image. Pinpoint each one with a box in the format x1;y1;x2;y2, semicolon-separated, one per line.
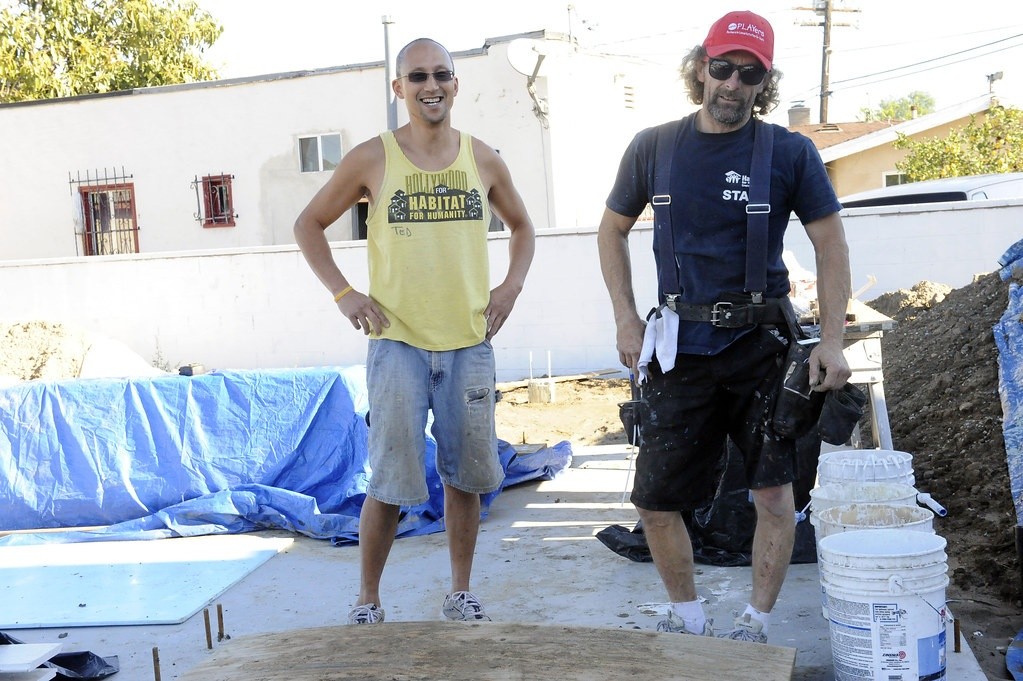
717;613;767;644
442;590;494;622
655;608;714;637
345;603;385;625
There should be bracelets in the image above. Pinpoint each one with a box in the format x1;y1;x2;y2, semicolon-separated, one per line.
334;285;352;303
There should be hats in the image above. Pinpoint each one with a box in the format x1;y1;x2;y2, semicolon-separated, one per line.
704;9;775;72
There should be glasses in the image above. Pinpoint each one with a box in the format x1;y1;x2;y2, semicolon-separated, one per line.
706;53;767;86
396;70;454;83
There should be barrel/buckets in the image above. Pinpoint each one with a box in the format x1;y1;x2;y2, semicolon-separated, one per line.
818;504;937;537
811;484;918;620
816;447;917;487
819;528;953;681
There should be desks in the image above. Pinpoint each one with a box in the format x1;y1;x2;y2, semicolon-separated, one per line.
802;280;898;451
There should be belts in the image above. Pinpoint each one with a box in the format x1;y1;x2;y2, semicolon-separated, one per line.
665;299;777;326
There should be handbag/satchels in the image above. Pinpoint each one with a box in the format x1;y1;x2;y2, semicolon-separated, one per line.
768;339;868;447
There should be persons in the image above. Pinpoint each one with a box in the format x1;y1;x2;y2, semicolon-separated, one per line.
598;10;852;643
294;39;535;624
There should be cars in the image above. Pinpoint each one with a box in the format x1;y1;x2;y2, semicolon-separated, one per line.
835;174;1023;209
